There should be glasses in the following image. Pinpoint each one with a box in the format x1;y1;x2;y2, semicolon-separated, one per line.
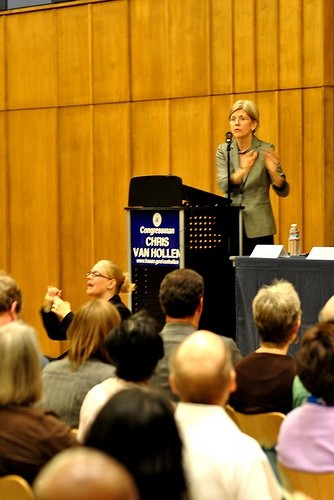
229;117;254;121
86;271;112;280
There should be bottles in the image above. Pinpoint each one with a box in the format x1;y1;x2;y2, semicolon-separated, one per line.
288;224;300;257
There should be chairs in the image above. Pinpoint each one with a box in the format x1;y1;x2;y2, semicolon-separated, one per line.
224;405;287;450
0;474;35;500
276;463;334;500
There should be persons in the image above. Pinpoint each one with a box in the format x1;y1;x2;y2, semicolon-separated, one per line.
0;259;333;500
216;100;289;257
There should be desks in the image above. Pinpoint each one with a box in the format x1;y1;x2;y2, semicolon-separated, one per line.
229;256;334;359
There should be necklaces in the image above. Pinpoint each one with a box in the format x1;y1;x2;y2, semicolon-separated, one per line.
238;150;250;155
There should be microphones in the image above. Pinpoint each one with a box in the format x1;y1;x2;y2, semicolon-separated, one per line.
225;132;233;143
